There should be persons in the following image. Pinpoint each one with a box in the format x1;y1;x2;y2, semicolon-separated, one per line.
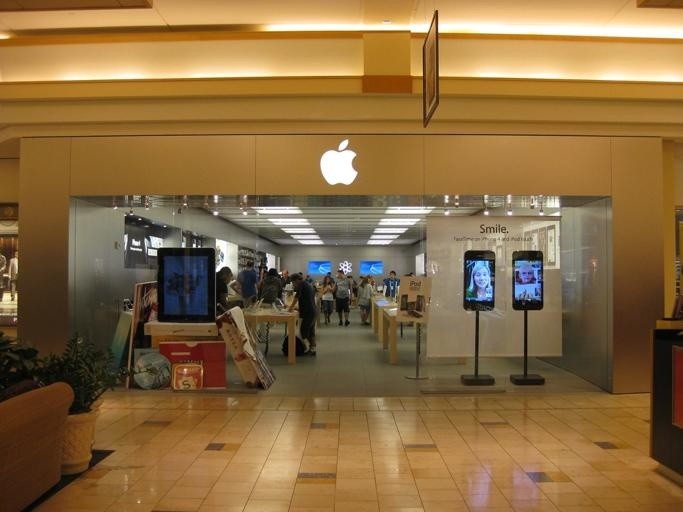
7;250;18;303
0;253;6;302
466;260;493;304
214;255;416;357
517;264;536;285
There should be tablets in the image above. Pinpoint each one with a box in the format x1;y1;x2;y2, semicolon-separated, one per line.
157;248;216;323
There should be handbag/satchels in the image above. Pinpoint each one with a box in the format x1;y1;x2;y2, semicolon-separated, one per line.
281;335;305;357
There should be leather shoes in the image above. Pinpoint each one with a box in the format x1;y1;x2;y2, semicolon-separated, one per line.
304;350;316;356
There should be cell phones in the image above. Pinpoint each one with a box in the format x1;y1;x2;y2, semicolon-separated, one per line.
463;250;495;312
512;250;543;311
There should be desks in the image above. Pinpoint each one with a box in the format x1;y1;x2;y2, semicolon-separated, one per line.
371;294;428;365
226;293;321;365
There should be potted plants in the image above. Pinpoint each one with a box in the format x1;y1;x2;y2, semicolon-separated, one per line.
34;330;162;476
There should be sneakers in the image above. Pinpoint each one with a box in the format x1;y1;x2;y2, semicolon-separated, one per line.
324;318;371;326
